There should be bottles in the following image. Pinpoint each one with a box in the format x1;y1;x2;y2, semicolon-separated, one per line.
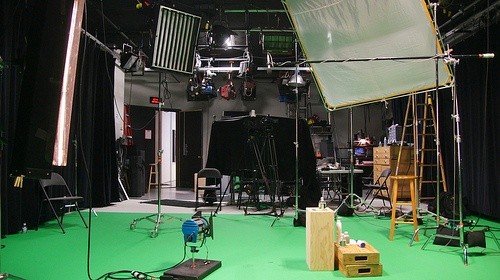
378;141;382;147
384;137;387;147
357;240;366;247
336;220;349;246
318;196;326;209
22;223;27;233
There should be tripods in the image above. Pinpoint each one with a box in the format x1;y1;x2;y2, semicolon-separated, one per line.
213;124;284;217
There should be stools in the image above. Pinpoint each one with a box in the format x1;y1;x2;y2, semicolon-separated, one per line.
390;175;419;241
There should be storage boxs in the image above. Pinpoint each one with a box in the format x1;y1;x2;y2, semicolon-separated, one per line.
306;208;335;270
334;241;382;277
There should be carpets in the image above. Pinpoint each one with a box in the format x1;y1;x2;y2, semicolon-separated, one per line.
142;199;220;209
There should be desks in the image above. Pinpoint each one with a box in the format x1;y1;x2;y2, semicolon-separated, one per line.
318;167;363;207
148;164;158;191
355;164;375;195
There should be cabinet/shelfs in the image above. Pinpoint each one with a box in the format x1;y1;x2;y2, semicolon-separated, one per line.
374;145;420;199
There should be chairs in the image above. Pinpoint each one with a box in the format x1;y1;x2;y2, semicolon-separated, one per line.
195;168;222;211
230;170;259;206
35;172;88;234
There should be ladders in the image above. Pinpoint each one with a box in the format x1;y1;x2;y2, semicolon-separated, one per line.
393;93;446;211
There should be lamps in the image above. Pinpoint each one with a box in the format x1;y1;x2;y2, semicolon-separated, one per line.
120;0;307;103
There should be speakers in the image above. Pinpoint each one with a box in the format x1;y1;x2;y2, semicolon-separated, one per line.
187;82;217;101
341;174;362;199
240;80;256;101
